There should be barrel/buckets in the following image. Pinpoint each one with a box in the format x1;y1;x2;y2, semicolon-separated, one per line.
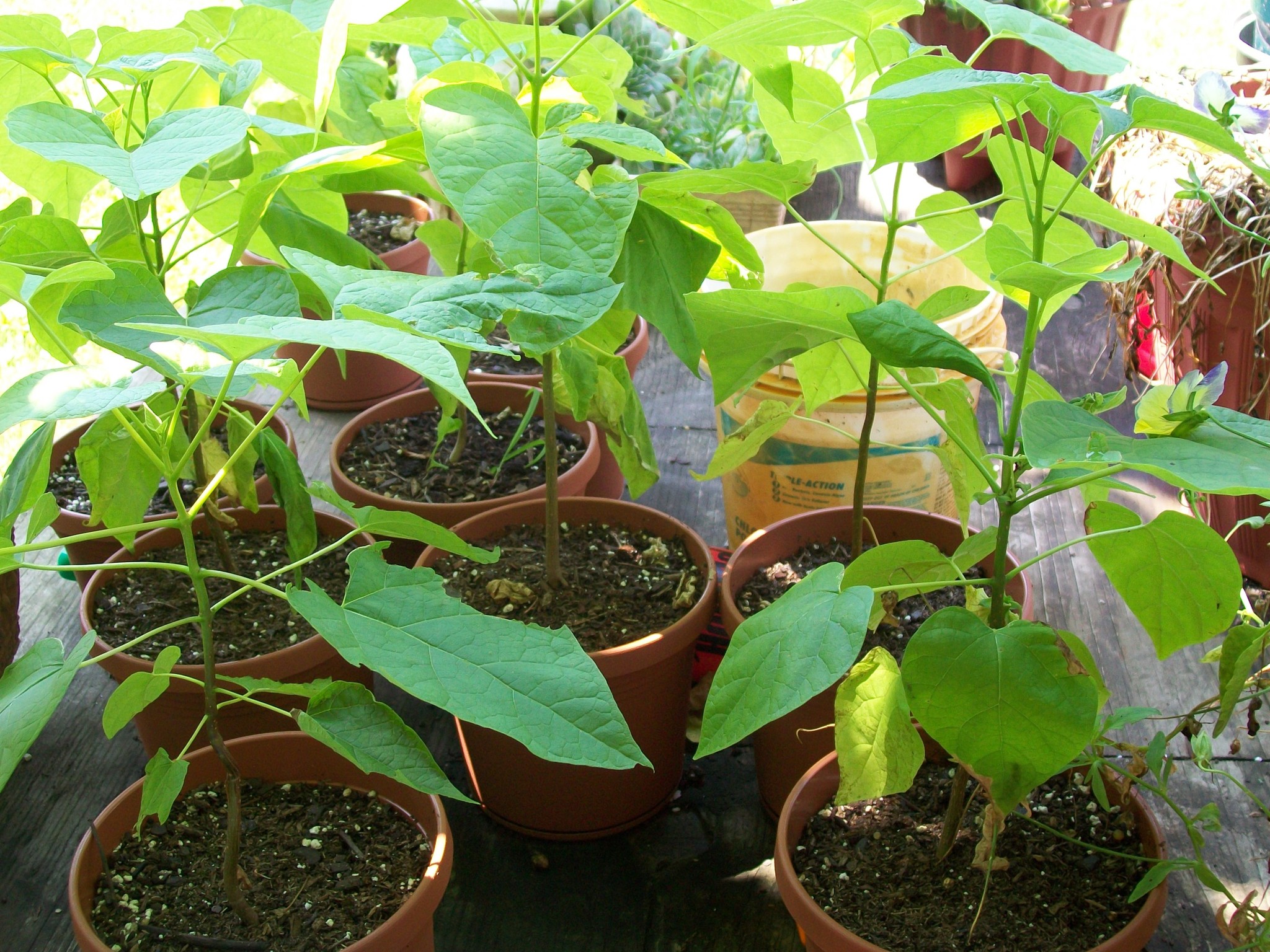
697;220;1008;554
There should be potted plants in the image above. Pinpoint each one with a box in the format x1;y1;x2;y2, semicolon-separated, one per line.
0;0;1270;952
